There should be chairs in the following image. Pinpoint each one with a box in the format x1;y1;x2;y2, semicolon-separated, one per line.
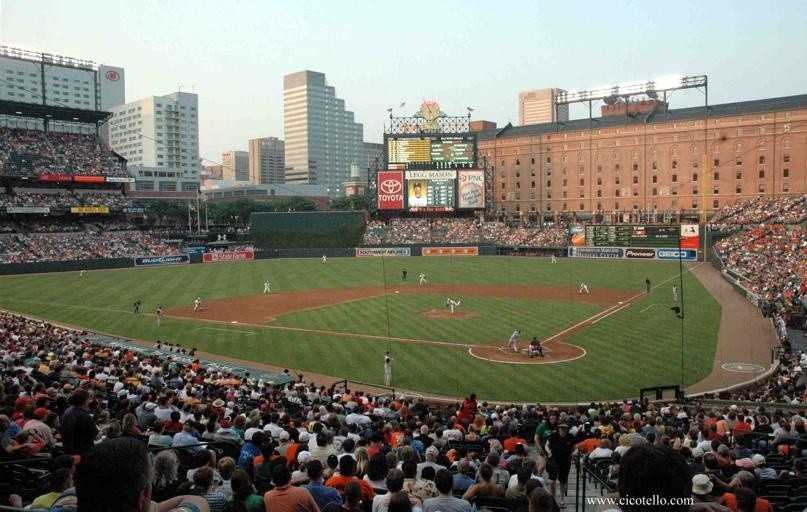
568;441;806;512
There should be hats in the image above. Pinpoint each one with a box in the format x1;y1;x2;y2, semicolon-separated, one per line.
54;455;81;468
63;383;74;389
279;431;290;440
118;389;129;397
299;432;311;442
14;397;32;404
692;473;713;496
145;403;157;411
735;454;766;469
46;387;58;393
212;398;225;407
32;408;51;416
298;451;312;464
